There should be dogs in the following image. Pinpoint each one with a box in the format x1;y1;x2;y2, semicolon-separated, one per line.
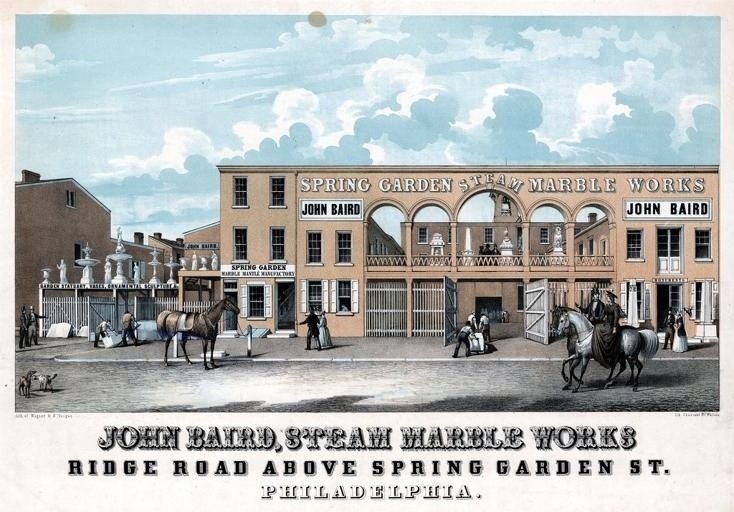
18;370;37;398
32;373;58;393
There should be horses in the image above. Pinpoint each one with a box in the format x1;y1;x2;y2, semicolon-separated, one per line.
156;295;241;370
556;309;659;394
550;303;656;384
